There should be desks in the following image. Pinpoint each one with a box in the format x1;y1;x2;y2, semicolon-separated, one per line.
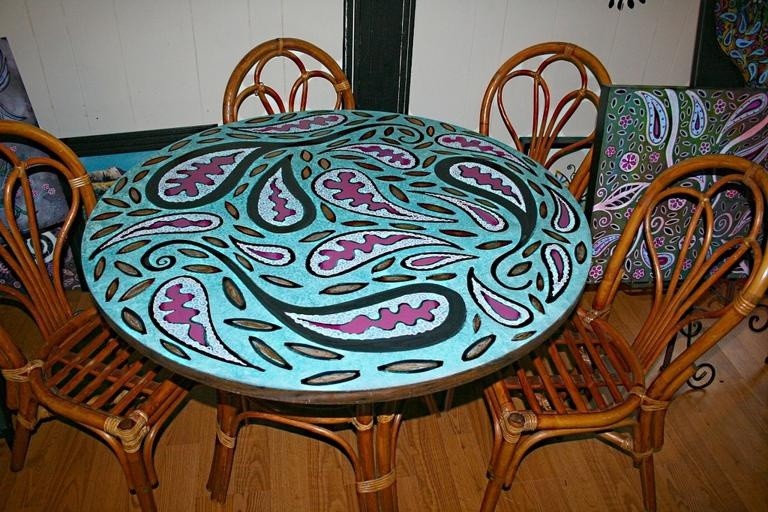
81;109;591;512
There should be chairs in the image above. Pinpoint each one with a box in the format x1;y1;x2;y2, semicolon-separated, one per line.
480;42;611;203
224;38;356;124
0;120;201;512
477;156;763;511
